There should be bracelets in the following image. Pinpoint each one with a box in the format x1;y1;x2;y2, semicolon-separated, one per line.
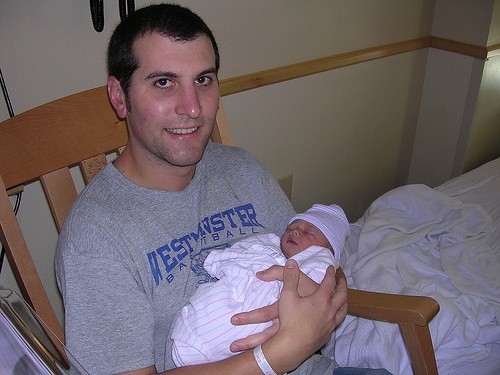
253;343;285;375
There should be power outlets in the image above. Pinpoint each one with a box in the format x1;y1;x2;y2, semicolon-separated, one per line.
275;171;292;205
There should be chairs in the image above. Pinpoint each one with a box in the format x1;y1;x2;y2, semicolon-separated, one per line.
0;85;441;375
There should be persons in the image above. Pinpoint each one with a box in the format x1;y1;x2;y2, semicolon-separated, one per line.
54;7;395;375
171;203;348;368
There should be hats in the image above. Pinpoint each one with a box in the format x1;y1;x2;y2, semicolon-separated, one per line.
288;203;350;262
